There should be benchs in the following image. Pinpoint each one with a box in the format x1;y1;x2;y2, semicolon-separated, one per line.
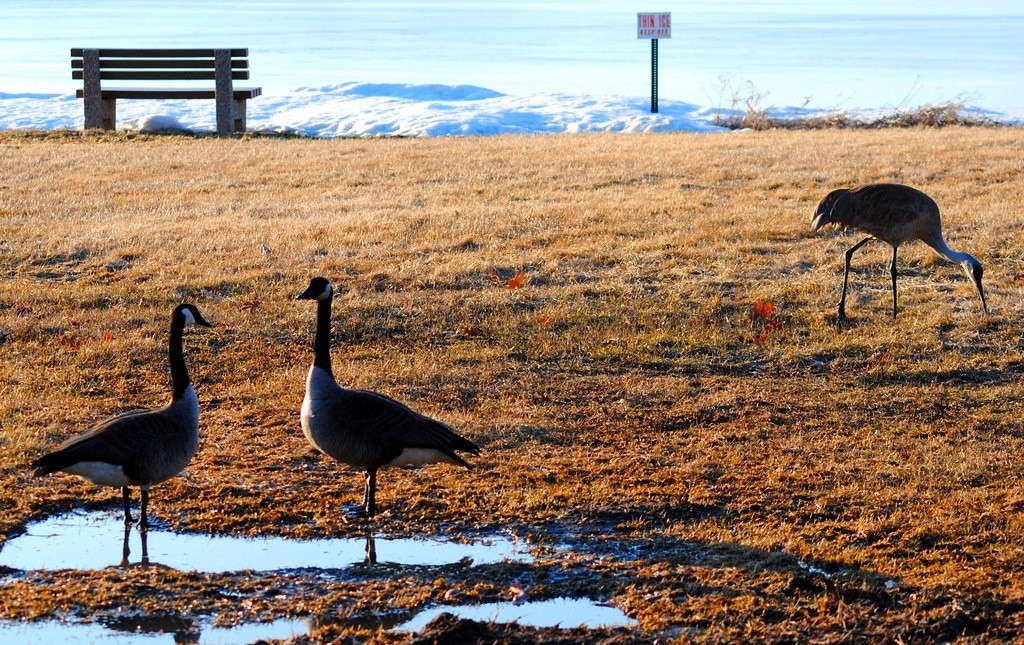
69;47;262;136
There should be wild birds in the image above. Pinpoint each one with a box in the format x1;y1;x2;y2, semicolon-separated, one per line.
808;183;987;323
25;304;213;526
295;278;483;520
